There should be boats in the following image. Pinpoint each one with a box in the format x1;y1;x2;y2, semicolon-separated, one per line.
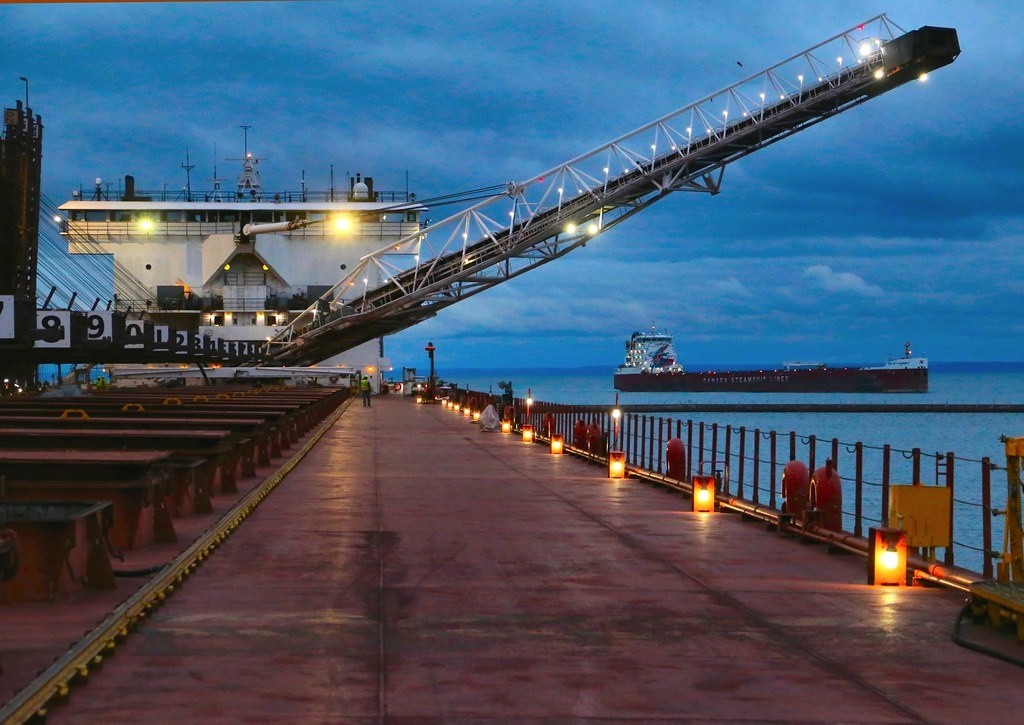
613;320;929;393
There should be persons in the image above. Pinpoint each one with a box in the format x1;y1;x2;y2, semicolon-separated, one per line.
360;375;371;407
96;376;108;390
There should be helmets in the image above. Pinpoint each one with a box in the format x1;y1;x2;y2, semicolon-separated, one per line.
364;375;369;378
